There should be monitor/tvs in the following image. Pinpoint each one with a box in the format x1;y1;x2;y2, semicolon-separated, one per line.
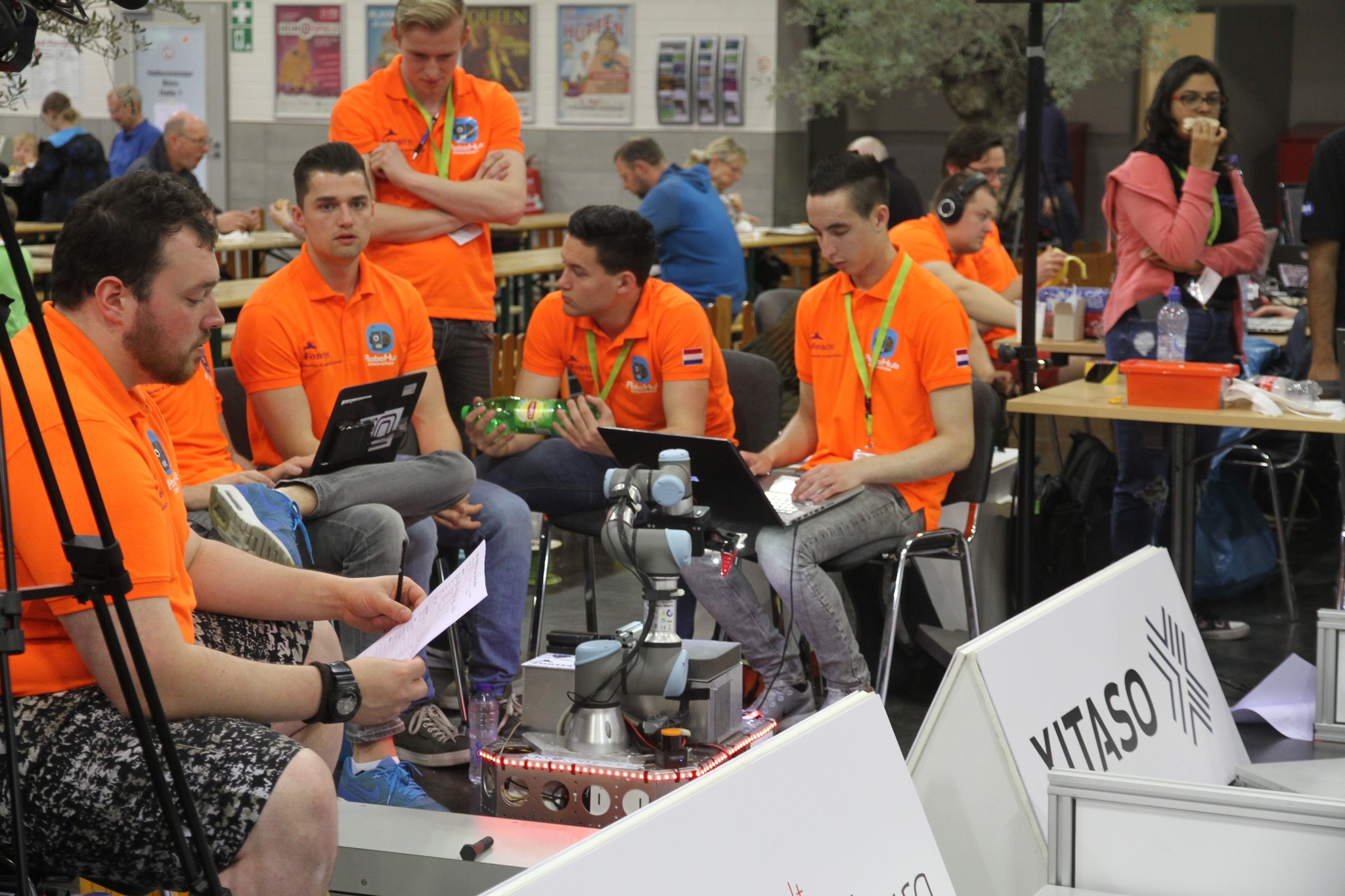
1278;181;1308;247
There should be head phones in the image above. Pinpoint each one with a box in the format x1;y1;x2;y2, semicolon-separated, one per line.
938;171;988;225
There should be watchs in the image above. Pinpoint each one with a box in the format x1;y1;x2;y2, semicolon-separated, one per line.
315;659;361;725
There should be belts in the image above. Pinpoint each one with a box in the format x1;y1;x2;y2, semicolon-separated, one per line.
1184;297;1232;310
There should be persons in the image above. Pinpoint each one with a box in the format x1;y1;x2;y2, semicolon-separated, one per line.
1104;55;1270;645
0;169;434;896
19;92;111;245
1;191;36;336
681;138;761;233
674;151;980;731
229;141;535;764
1014;77;1084;251
8;132;38;179
889;169;1025;398
614;136;749;323
122;110;263;232
329;0;528;656
940;128;1071;390
440;199;739;716
105;84;163;181
120;171;477;814
847;135;926;230
1299;124;1345;567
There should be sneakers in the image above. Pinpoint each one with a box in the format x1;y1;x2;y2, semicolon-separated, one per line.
393;704;470;766
208;480;313;567
745;680;818;734
338;756;449;812
819;686;876;708
466;683;529;739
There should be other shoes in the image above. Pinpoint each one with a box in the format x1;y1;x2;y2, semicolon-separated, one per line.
1197;620;1251;641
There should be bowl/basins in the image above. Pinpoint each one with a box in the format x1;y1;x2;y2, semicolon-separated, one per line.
1119;359;1240;411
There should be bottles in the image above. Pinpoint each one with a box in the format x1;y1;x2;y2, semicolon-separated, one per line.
660;729;682;752
1244;374;1323;410
468;682;498;785
461;396;600;436
1157;286;1188;362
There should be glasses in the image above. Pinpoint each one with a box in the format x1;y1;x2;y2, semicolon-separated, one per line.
178;136;212;148
965;163;1012;181
1172;92;1228;110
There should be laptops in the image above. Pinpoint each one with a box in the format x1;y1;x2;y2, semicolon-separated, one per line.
597;426;866;528
303;370;428;476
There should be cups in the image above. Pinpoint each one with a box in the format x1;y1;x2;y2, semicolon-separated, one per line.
1014;300;1047;343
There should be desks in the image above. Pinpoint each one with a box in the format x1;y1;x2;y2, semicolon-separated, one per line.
992;326;1313;360
1006;373;1344;591
15;217;818;367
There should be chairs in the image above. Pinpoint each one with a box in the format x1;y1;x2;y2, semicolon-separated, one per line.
212;227;1344;713
